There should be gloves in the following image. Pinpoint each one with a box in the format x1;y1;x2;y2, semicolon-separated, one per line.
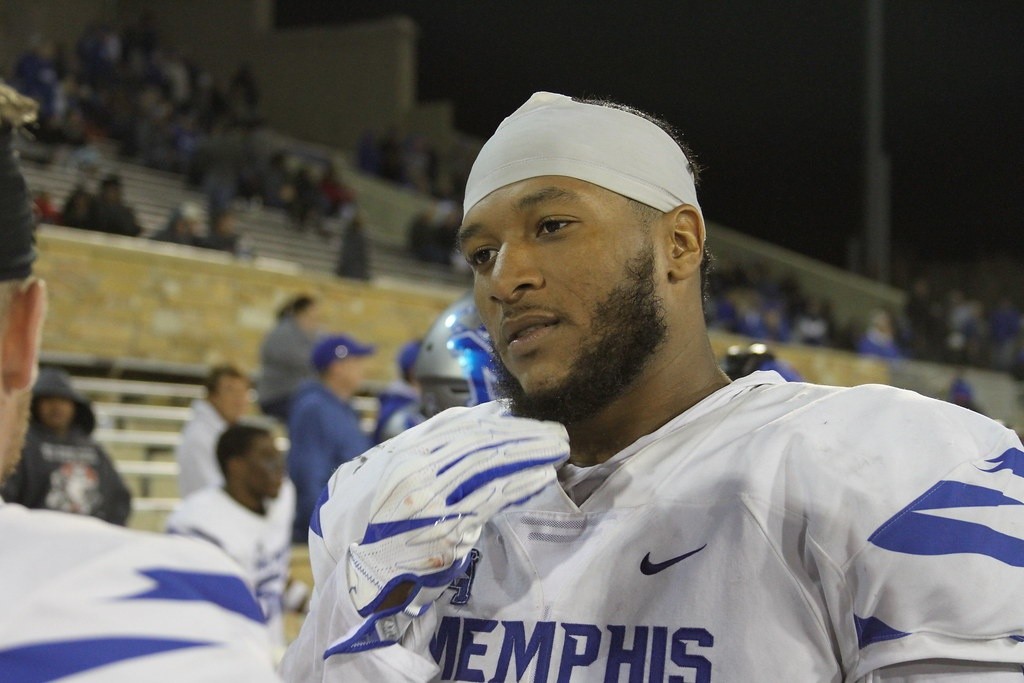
319;398;570;660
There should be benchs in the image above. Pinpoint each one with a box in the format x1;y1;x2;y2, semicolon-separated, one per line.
0;33;1024;646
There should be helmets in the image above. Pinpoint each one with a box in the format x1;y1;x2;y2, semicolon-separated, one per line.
410;291;504;416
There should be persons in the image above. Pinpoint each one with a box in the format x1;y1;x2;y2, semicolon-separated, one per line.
1;372;129;525
0;114;281;683
285;337;375;543
714;262;1024;414
166;424;296;645
12;23;466;281
375;342;422;443
259;297;317;419
174;365;251;495
278;92;1024;683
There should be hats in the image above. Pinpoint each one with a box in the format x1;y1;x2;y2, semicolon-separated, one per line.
313;336;372;371
